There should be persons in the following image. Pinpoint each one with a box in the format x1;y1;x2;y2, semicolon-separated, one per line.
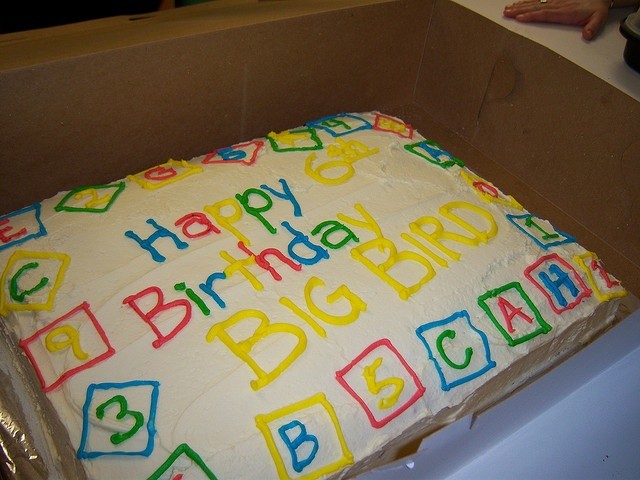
503;0;639;40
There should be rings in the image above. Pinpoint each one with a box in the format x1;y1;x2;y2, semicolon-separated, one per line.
540;0;547;4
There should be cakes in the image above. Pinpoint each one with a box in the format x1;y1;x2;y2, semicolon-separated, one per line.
0;109;626;479
